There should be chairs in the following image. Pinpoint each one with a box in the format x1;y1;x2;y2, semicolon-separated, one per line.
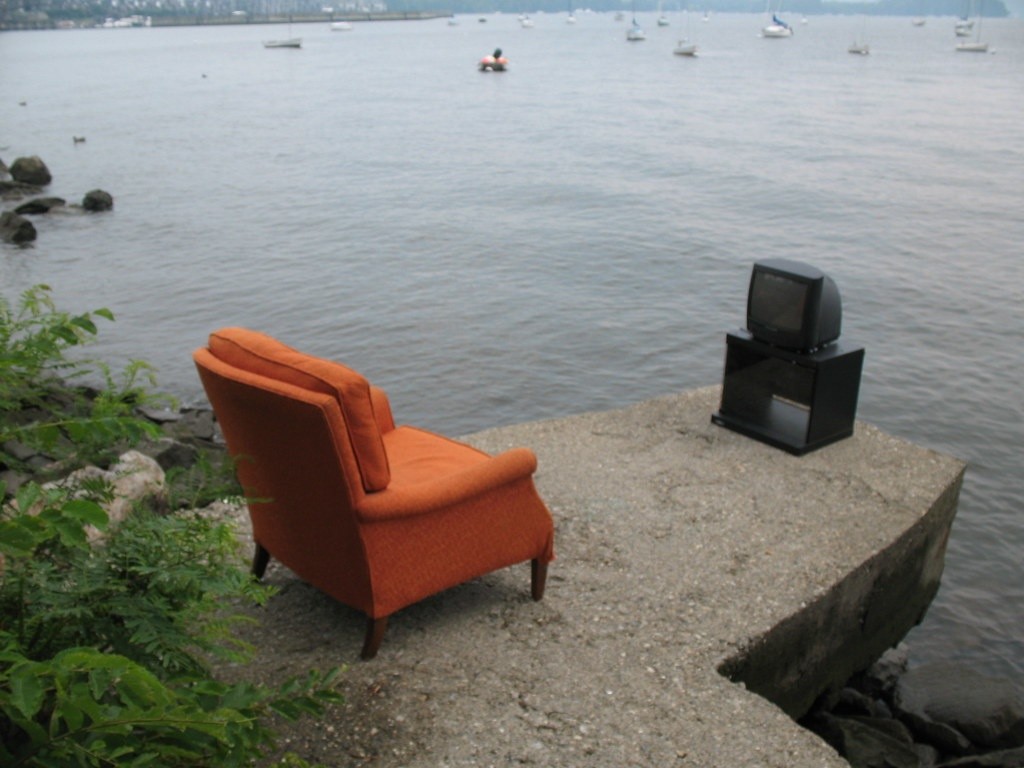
192;325;557;661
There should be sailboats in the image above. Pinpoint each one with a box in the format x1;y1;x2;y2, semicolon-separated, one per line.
628;1;645;40
761;0;793;37
656;0;669;27
675;3;695;55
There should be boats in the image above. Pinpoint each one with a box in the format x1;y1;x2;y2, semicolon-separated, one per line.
954;0;988;51
446;0;625;29
910;17;926;25
848;40;869;54
262;37;303;48
330;21;352;31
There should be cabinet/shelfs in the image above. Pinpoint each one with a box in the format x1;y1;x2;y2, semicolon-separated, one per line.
711;329;866;456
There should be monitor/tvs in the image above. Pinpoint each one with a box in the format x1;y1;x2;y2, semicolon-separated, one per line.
746;258;841;355
